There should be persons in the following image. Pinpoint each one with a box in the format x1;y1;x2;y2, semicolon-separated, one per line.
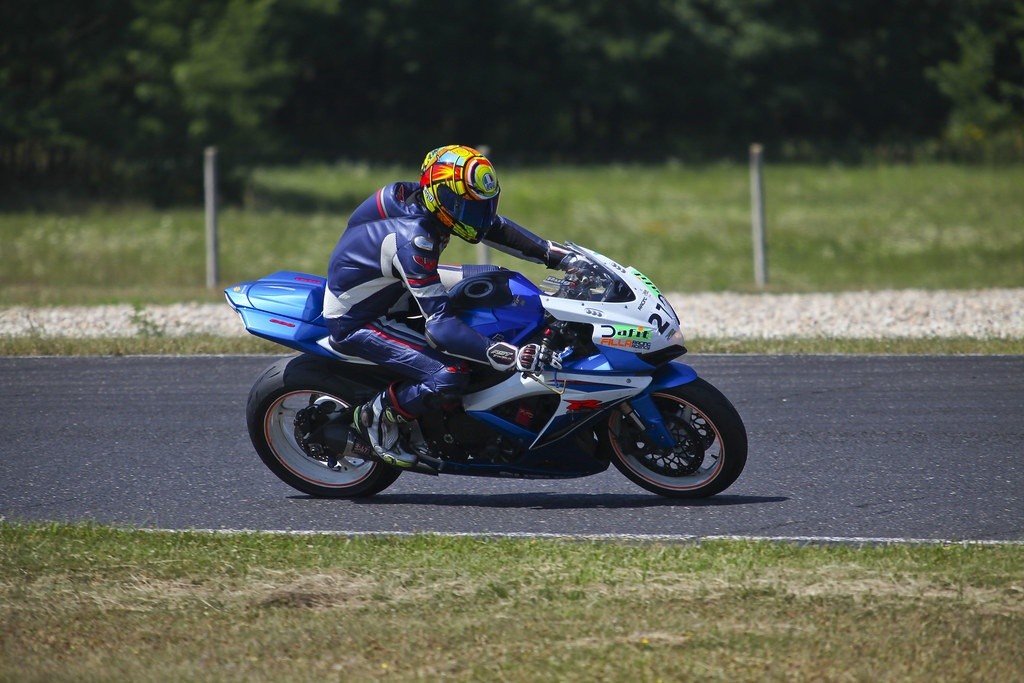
323;145;609;467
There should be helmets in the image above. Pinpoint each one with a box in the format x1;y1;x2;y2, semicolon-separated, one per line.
421;145;500;244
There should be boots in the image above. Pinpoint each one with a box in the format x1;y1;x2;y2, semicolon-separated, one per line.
353;380;417;468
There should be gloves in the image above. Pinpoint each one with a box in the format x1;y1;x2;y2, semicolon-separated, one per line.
486;341;563;375
547;240;593;271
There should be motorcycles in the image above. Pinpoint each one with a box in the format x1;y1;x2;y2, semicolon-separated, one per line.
222;242;749;503
